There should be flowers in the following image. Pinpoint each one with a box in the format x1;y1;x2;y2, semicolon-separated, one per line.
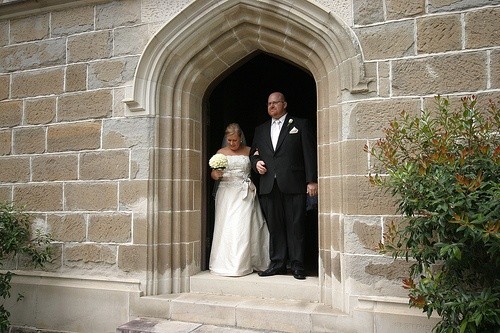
208;153;228;170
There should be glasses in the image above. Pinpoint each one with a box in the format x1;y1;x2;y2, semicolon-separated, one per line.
265;100;283;106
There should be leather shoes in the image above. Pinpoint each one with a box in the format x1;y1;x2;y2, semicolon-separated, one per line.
258;266;287;276
292;262;307;279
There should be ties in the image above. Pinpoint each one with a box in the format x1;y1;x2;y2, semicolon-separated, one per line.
272;120;280;152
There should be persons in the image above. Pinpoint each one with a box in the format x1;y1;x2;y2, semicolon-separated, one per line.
250;92;319;280
208;121;270;278
